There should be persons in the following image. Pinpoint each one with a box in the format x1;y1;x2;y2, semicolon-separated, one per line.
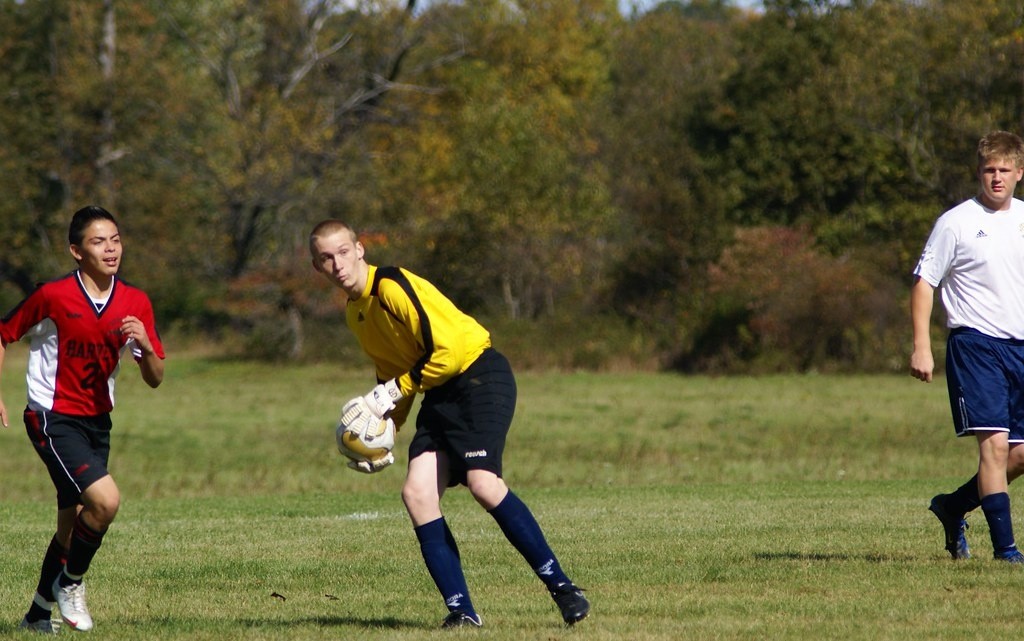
309;220;594;632
0;206;166;639
909;131;1024;564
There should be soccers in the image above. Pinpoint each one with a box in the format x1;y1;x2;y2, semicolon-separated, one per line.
336;416;398;464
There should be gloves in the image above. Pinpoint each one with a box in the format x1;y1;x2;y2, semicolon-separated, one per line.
339;383;393;440
348;451;394;472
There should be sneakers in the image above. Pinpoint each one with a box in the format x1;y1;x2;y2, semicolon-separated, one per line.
442;611;483;630
929;494;970;560
52;572;93;632
19;615;56;638
551;583;591;625
993;546;1024;564
